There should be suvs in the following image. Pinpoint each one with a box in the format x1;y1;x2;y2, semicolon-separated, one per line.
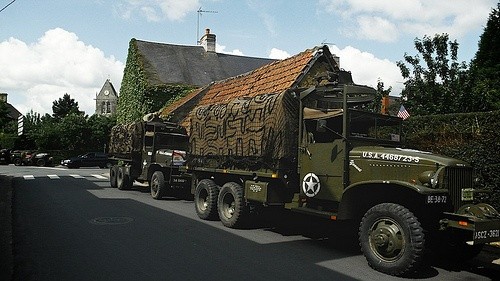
67;151;107;169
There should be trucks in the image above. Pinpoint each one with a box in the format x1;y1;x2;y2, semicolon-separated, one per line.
178;83;500;277
107;120;194;201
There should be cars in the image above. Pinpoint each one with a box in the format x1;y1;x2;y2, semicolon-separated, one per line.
0;149;53;166
